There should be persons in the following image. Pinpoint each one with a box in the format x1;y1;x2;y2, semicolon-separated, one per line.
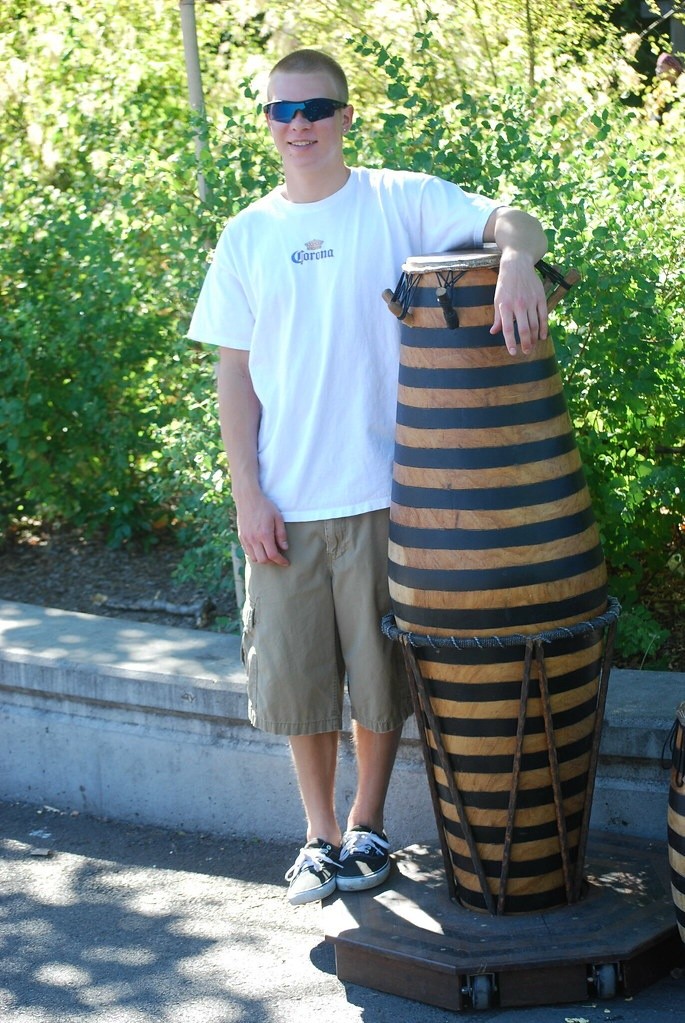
183;50;549;905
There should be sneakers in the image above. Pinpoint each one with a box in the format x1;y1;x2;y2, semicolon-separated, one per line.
336;825;391;891
284;837;342;906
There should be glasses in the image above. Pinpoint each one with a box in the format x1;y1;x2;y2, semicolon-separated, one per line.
263;98;348;123
